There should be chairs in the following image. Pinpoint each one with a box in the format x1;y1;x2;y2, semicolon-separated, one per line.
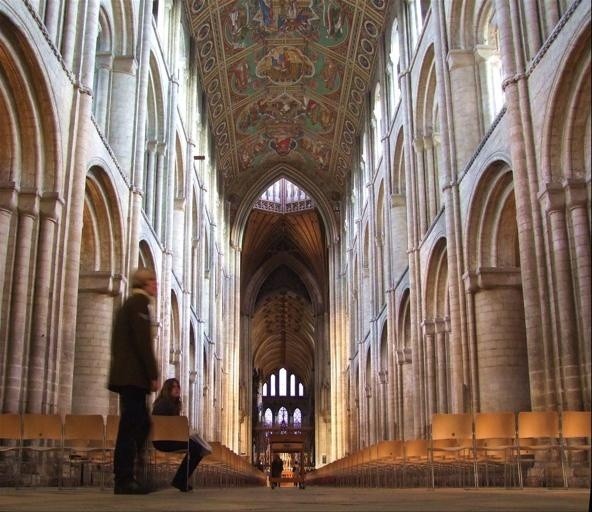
306;410;591;493
0;409;267;491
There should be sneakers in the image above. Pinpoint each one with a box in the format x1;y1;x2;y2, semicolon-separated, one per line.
169;477;193;493
111;477;152;496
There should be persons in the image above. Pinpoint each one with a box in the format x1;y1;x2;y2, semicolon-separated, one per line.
108;267;161;495
152;377;205;492
272;454;284;488
292;460;300;486
